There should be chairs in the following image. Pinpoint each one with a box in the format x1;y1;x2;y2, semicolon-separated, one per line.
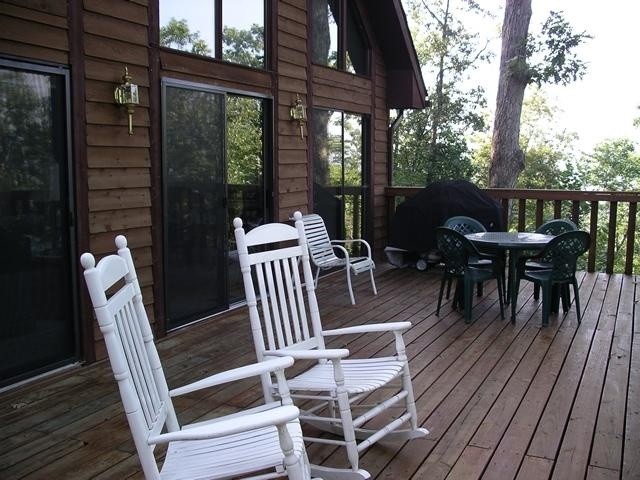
435;226;505;323
514;219;579;308
80;235;324;480
288;213;378;306
444;216;508;311
514;231;592;327
233;210;431;480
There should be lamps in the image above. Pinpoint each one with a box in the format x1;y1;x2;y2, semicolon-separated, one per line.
290;92;307;140
114;65;140;136
